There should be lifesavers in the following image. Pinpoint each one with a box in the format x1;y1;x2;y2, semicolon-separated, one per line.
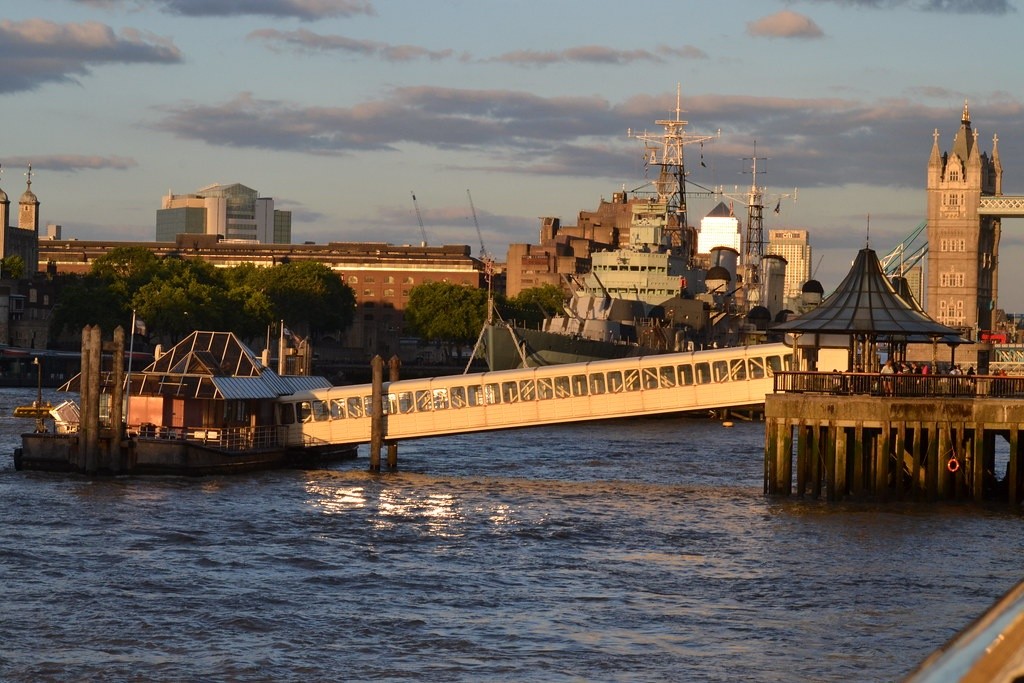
946;458;959;474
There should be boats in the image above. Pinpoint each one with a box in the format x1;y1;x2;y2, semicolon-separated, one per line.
481;82;799;395
13;314;358;470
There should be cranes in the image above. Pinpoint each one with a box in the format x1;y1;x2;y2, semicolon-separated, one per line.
409;190;427;243
465;189;488;259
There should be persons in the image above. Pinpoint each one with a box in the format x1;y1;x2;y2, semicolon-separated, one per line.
856;362;863;372
921;364;927;374
833;369;851;392
900;362;919;394
966;367;978;397
950;365;963;397
880;360;894;396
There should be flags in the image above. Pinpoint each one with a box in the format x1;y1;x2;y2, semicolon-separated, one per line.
134;320;146;336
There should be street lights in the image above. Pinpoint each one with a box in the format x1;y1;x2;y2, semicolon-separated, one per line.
31;357;42;413
